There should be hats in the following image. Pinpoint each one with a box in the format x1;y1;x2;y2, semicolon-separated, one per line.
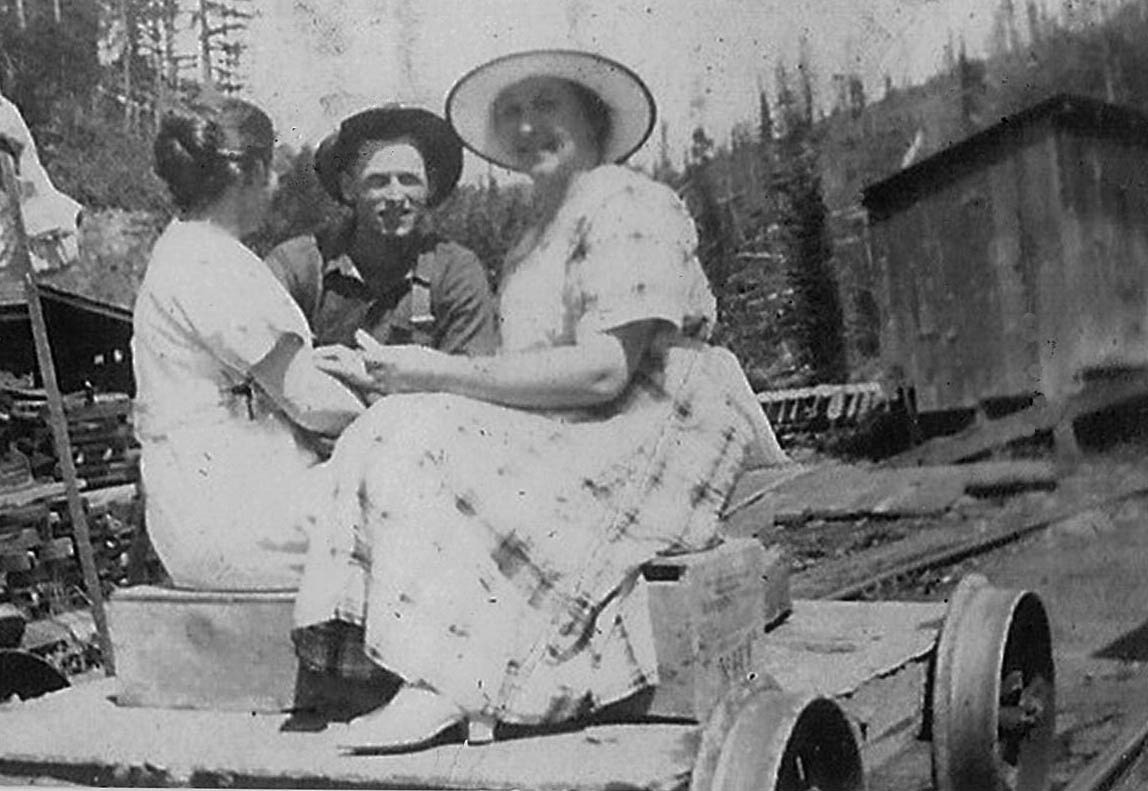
314;105;463;208
447;49;658;172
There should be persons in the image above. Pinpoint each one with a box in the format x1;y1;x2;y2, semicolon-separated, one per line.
130;96;367;592
264;101;497;357
289;50;821;753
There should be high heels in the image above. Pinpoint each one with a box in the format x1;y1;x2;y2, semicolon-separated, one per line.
335;674;496;750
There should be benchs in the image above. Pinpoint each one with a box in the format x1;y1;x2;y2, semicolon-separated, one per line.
97;468;821;722
